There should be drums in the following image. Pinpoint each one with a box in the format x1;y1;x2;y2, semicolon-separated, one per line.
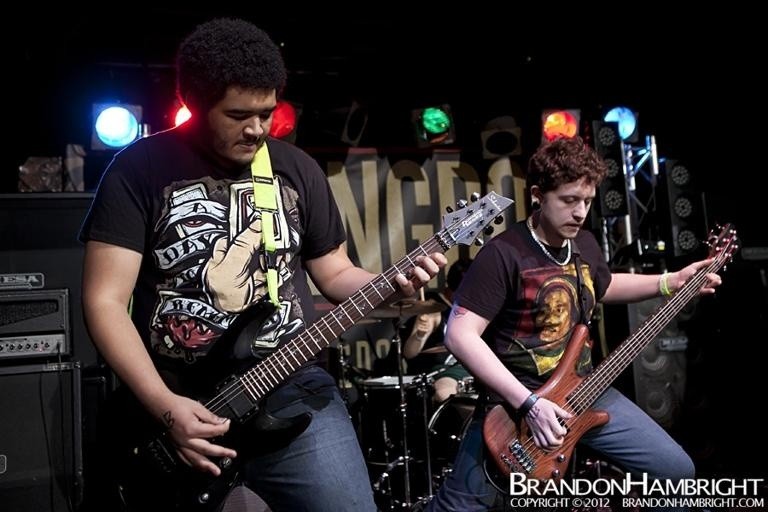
440;393;478;470
355;375;436;467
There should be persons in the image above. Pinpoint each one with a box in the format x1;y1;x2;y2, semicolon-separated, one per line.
402;258;476;400
425;134;722;512
508;274;592;377
76;15;451;510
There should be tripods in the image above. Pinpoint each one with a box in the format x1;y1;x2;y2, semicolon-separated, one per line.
646;133;660;176
407;411;437;512
371;359;430;495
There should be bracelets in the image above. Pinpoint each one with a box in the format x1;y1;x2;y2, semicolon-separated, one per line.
658;271;673;297
414;333;421;340
518;392;540;417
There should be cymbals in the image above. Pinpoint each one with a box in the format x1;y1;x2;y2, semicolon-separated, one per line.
369;300;440;318
420;344;450;354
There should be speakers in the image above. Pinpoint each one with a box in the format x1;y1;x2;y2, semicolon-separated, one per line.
0;357;85;512
661;156;714;258
590;119;629;219
604;295;689;425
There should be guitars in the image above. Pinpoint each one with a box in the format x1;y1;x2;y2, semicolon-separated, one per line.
107;192;514;512
482;223;739;493
